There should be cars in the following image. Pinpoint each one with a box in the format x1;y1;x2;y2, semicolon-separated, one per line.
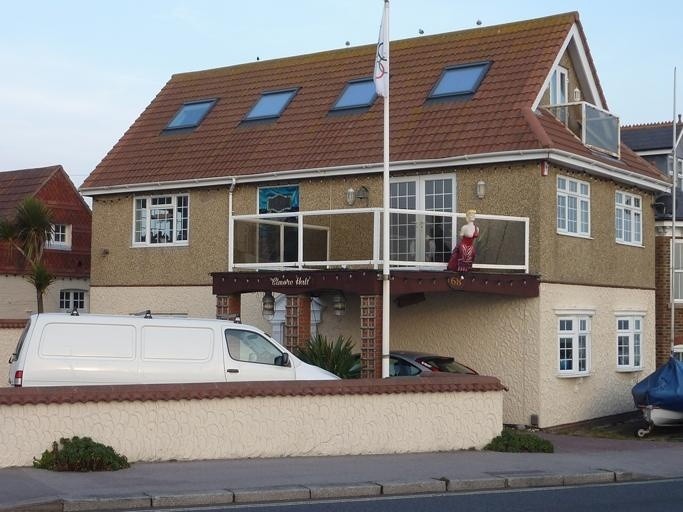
348;350;478;381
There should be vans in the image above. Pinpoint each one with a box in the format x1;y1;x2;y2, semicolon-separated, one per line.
4;309;341;388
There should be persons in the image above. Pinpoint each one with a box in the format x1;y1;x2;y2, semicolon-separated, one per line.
442;209;481;273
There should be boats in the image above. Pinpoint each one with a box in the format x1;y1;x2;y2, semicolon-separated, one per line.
632;404;682;440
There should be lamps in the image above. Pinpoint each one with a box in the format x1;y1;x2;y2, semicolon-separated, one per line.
574;86;580;103
261;292;276;322
356;185;369;197
332;295;346;316
347;187;354;204
477;180;486;199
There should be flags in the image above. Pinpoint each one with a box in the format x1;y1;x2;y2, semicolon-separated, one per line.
372;3;389;97
